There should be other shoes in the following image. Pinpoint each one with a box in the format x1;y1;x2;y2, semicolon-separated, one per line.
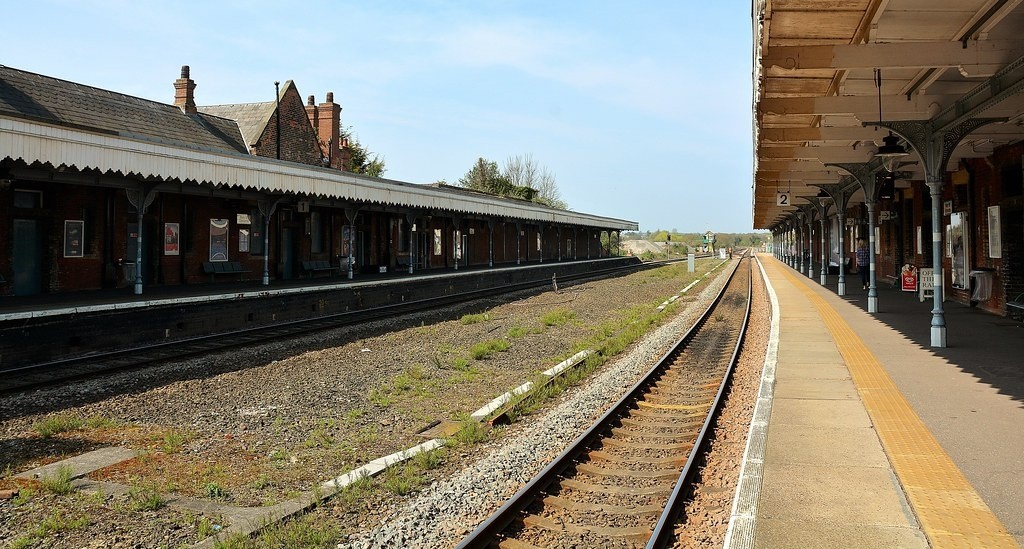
863;286;866;289
867;282;869;287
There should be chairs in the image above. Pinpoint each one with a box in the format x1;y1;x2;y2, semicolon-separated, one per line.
302;261;337;278
203;261;254;282
397;255;424;273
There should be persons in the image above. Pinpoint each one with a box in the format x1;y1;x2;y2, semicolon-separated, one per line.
857;238;870;291
